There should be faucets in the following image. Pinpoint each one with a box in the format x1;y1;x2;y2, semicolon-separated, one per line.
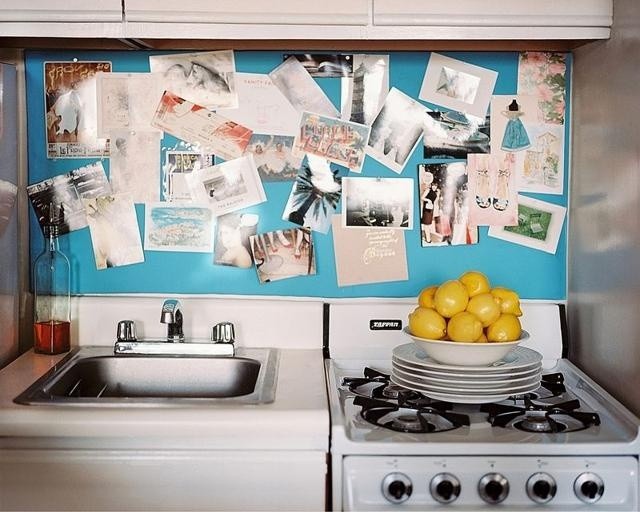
159;299;186;343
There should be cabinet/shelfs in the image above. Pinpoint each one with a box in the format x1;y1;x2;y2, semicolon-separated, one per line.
0;0;139;54
124;1;614;55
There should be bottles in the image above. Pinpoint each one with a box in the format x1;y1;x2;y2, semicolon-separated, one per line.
33;202;73;356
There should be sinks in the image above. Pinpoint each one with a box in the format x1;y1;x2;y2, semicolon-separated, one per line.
11;345;281;405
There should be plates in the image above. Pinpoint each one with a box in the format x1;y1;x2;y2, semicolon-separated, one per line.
391;341;542;405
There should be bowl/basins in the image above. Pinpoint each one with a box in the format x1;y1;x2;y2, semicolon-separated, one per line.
403;327;533;364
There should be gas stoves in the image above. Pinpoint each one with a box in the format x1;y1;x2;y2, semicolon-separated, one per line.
328;357;640;511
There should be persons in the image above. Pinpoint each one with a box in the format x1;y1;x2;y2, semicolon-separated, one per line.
250;227;310;270
46;80;83;143
248;139;287;176
218;218;251;269
299;114;362;165
476;154;510;210
422;171;469;244
501;98;531;163
111;138;137;191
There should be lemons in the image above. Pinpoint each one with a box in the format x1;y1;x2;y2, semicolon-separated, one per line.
408;306;446;340
447;311;483;343
489;288;522;317
459;272;489;298
488;314;521;342
434;281;470;318
466;294;502;328
476;333;487;343
418;287;436;308
